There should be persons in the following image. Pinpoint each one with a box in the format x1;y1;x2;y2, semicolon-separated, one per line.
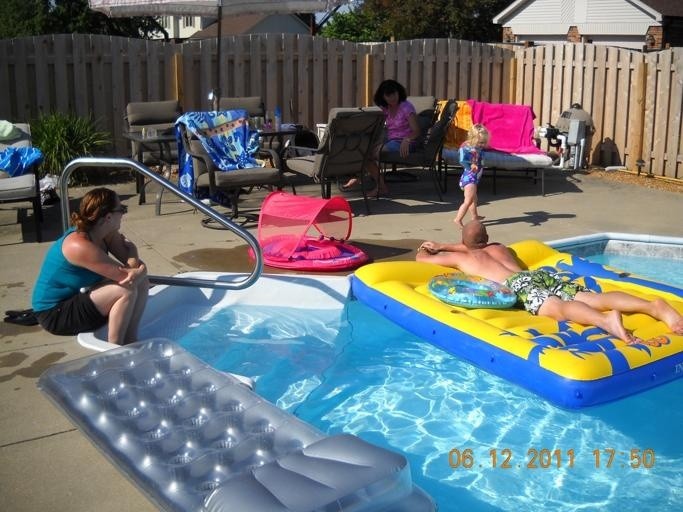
453;125;490;232
344;80;421;199
415;221;683;345
31;188;150;346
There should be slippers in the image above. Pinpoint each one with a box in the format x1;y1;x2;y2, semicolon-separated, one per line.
5;308;33;317
4;312;37;327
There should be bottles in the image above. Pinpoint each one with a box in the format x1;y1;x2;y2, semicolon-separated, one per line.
254;117;259;130
259;117;263;131
274;105;281;133
142;126;160;139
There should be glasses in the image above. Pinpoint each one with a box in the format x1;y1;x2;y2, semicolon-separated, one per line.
108;204;130;216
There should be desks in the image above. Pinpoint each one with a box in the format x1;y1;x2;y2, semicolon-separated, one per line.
122;118;296;218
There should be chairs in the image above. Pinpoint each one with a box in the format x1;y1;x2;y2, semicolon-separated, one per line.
121;97;183;206
407;95;435;116
287;105;385;214
378;99;459;201
210;94;268;119
0;121;43;241
416;108;441;139
176;110;285;231
438;101;553;198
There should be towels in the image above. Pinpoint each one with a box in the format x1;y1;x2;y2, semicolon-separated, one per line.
0;147;44;177
175;108;263;208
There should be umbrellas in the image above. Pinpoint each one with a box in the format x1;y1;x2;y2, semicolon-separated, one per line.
85;0;338;113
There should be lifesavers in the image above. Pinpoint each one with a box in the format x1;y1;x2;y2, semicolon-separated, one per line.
249;235;369;271
429;272;518;310
